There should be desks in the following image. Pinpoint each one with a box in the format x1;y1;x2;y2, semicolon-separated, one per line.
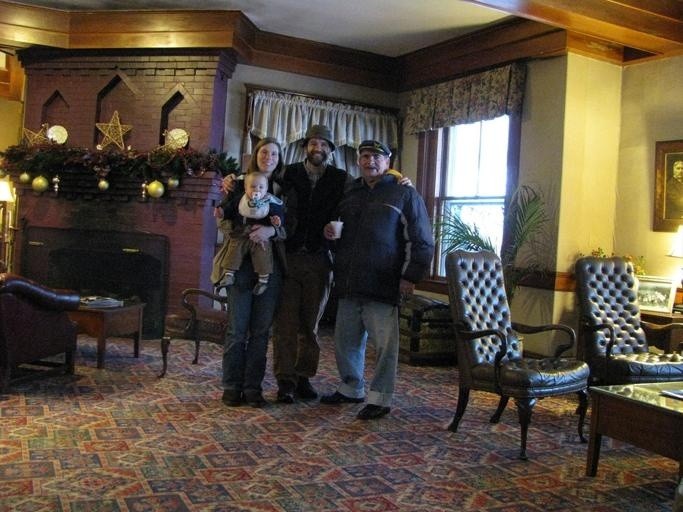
66;302;147;369
586;382;683;484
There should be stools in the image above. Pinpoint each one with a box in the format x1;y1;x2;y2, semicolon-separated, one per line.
400;293;450;351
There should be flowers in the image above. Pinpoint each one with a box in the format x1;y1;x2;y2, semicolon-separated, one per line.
576;247;648;276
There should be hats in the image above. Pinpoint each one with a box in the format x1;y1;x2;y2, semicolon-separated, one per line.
358;140;392;157
301;125;335;151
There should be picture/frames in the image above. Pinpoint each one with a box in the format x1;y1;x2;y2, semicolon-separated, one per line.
635;275;678;314
653;139;683;232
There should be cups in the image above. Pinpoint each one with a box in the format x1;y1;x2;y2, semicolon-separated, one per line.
330;221;344;238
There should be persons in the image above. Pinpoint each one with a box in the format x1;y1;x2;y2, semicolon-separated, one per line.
644;292;667;305
665;160;683;218
220;124;416;404
213;171;290;295
323;141;435;419
217;137;296;407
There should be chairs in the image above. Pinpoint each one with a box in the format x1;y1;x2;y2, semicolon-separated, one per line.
158;286;228;379
444;250;590;460
0;272;81;394
575;256;683;415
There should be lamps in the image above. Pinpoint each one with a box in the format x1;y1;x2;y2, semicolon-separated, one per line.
0;181;14;201
666;226;683;292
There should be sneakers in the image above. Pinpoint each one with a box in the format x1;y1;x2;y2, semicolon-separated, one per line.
298;379;317;398
252;283;268;295
358;404;390;420
277;384;296;403
245;393;267;407
321;390;364;403
220;275;235;287
222;389;242;406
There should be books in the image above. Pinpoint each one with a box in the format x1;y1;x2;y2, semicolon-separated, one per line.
661;390;683;401
